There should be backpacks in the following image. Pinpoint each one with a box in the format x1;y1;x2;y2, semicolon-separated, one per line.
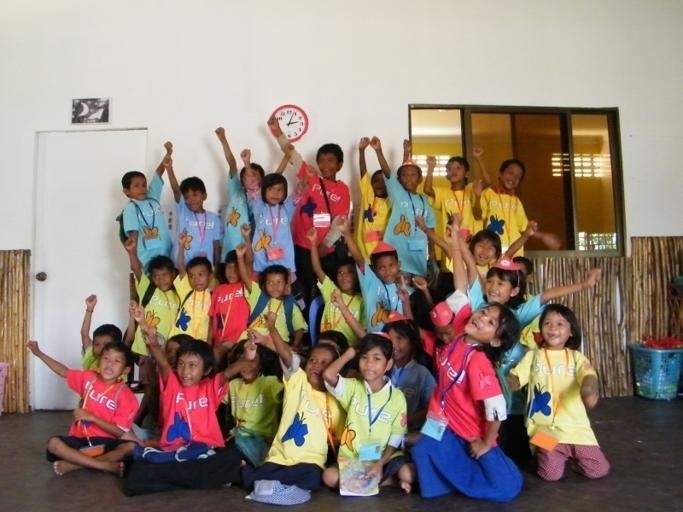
118;197;162;246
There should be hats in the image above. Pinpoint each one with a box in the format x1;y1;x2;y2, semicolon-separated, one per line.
493;257;522;287
370;242;395;255
429;302;454;326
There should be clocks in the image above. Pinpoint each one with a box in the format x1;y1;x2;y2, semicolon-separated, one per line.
270;105;308;140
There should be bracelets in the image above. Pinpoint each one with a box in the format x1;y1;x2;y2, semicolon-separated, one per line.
346;315;353;324
86;309;93;312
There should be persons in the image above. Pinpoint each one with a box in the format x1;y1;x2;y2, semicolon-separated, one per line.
410;225;519;501
451;213;602;459
470;158;561;260
506;303;610;481
25;341;139;478
336;216;433;332
305;226;365;369
371;135;436;287
356;137;391;266
247;329;360;382
415;215;537;280
115;142;172;275
168;232;213;342
397;276;472;357
215;127;294;284
424;145;492;272
133;306;194;441
124;325;246;496
209;224;258;372
80;296;135;371
234;242;308;374
241;148;315;311
240;312;348;492
512;256;543;350
122;235;180;387
323;333;416;495
267;116;350;322
163;156;222;279
331;289;436;445
218;339;284;467
